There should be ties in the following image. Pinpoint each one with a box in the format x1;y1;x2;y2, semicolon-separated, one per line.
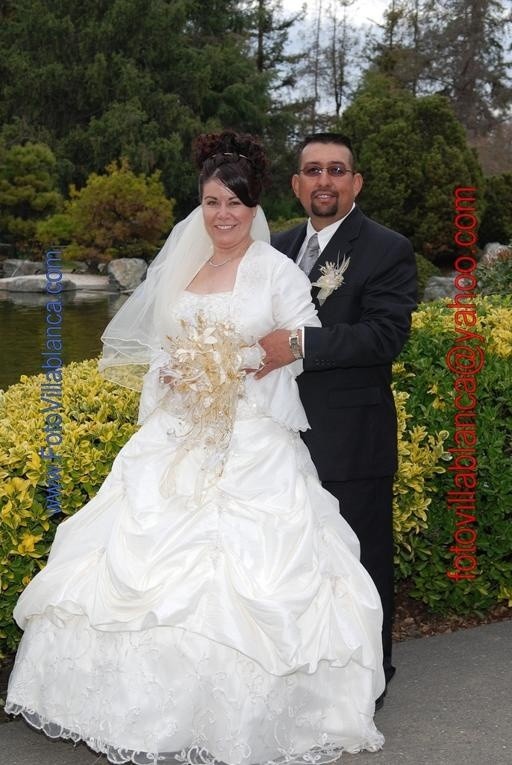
296;232;322;278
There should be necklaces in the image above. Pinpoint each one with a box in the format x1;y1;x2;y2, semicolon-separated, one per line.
210;248;245;268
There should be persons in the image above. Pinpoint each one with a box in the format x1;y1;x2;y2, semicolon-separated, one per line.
173;131;423;721
4;133;387;765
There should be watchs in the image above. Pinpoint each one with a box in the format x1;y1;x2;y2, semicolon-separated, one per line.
287;329;303;359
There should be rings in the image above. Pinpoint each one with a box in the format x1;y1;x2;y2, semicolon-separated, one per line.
260;361;266;367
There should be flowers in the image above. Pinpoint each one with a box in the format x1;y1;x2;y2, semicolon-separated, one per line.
312;250;352;307
156;306;253;501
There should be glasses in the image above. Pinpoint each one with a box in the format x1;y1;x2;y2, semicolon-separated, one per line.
296;163;357;180
